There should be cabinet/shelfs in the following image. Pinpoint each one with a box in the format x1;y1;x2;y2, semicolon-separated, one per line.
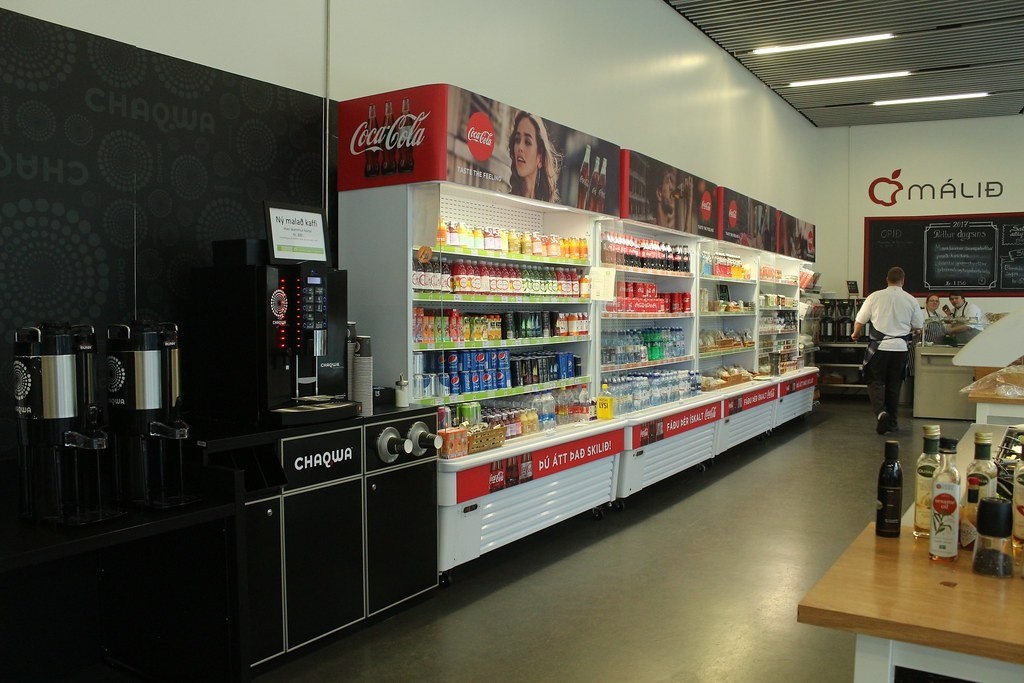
336;181;821;568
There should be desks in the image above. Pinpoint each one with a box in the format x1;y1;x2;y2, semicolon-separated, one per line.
793;423;1023;683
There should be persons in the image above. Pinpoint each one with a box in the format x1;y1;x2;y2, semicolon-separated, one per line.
920;292;989;343
506;111;563;205
851;266;924;434
641;167;694;233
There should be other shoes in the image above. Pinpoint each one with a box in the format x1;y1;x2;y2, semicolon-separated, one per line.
876;411;888;434
887;422;899;432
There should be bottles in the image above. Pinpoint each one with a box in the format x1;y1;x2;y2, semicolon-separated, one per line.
786;379;796;395
876;441;903;537
596;384;614;419
640;417;663;447
958;478;981;551
600;369;701;415
728;394;742;416
1012;436;1024;539
481;384;590;440
364;97;414;178
794;228;802;259
913;425;940;538
700;252;742;279
577;144;607;213
490;452;533;493
928;437;961;563
601;231;690;272
601;327;685;364
395;375;409;408
972;497;1014;578
560;312;588;336
412;217;590;298
966;432;998;497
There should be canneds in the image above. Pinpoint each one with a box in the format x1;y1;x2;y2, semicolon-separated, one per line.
413;348;512;400
509;350;581;387
640;346;648;362
605;281;692;313
438;401;483;431
493;310;562;340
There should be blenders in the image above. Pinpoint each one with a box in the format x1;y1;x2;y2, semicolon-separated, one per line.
14;323;109;525
106;318;192;506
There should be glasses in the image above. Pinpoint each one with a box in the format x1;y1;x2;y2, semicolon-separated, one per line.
928;300;939;303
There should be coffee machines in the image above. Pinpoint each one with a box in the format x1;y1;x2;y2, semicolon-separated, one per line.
192;201;361;425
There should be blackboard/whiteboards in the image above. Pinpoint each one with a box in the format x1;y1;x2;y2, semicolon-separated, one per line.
863;212;1024;298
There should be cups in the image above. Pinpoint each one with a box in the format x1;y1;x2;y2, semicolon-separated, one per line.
347;321;374;417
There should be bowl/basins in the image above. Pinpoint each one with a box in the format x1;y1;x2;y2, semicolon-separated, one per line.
821;292;836;299
